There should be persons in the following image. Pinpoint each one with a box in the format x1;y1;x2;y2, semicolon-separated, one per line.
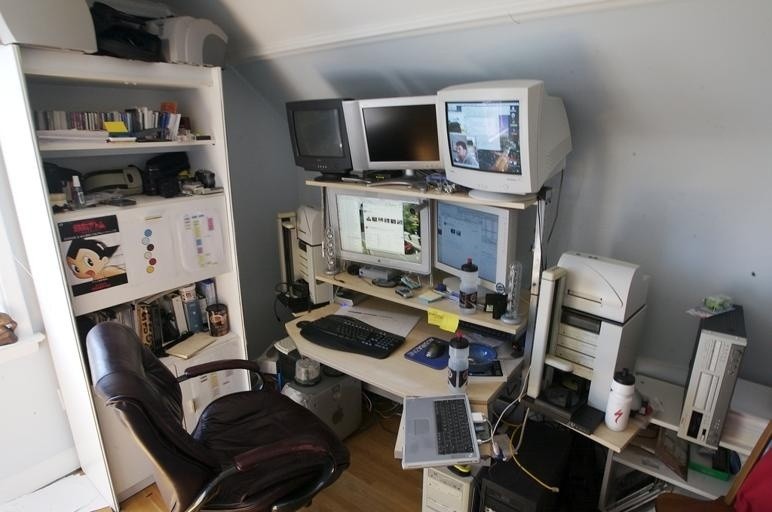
408;208;420;234
456;141;479;167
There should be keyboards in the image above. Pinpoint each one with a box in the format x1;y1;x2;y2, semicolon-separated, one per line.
403;393;480;469
300;313;405;359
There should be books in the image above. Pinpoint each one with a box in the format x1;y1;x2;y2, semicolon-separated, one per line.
35;107;181;141
605;471;669;512
90;293;216;360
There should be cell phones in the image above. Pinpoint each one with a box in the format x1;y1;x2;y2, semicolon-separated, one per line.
395;287;414;298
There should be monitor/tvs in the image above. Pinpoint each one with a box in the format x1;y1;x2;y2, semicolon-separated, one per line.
433;199;518;305
327;188;432;282
285;99;352;182
435;78;571;203
342;95;444;193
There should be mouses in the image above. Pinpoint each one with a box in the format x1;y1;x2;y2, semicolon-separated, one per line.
425;341;445;358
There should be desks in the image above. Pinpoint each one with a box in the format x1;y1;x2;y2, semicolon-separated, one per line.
284;295;534;512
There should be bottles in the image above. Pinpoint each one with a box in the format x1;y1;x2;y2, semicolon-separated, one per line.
605;368;637;432
68;174;89;210
446;331;470;393
458;257;478;314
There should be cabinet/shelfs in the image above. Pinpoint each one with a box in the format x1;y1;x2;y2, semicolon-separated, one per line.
595;407;772;512
1;41;251;512
306;177;541;334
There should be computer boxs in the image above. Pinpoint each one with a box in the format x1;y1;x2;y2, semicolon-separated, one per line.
422;460;488;512
281;366;363;441
480;422;570;512
677;299;747;453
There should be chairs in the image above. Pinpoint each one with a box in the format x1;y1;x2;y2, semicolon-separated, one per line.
83;319;354;511
651;418;772;509
